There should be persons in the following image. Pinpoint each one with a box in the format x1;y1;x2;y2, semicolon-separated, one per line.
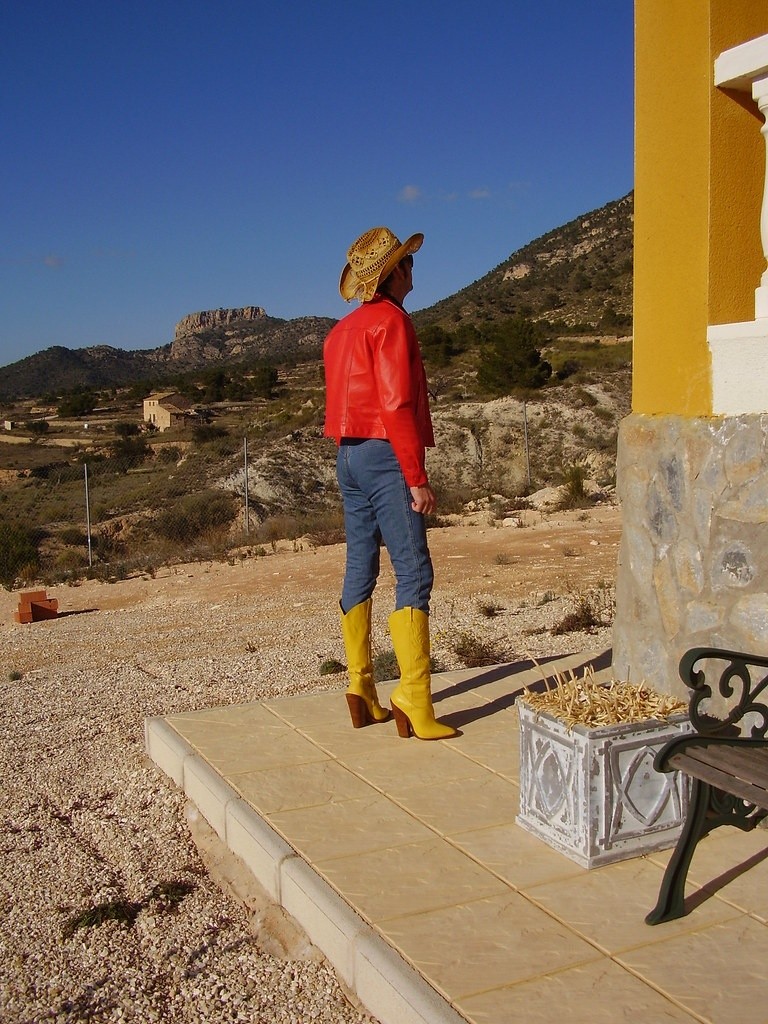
321;227;459;741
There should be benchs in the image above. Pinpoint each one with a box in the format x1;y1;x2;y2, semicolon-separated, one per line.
644;648;767;926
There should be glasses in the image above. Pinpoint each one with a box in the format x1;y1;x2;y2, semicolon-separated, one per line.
400;255;413;268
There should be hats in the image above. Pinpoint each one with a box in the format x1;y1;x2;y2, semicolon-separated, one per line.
339;227;425;304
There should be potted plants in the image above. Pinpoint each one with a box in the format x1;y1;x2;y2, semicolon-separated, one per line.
515;649;698;870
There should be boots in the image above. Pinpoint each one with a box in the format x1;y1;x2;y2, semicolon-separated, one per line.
338;596;389;728
388;605;458;739
